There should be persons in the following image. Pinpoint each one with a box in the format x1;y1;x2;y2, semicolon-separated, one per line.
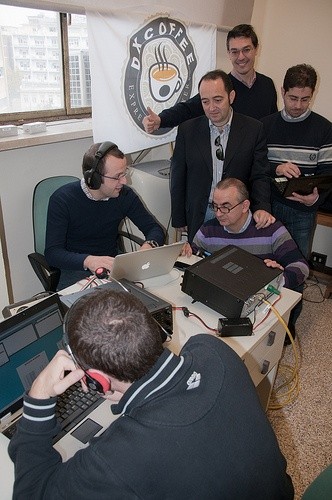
43;142;166;292
8;290;294;500
143;23;332;346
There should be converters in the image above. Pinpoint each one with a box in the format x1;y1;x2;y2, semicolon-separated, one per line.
218;317;253;337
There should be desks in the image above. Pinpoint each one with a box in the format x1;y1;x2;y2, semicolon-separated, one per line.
0;253;303;499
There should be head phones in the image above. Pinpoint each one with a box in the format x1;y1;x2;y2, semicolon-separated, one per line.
62;292;110;396
84;141;118;190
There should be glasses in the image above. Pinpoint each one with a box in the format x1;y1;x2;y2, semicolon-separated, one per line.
215;135;225;161
100;168;130;180
285;93;312;104
229;46;254;57
212;199;246;213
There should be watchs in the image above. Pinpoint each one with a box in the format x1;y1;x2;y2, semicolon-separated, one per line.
144;240;159;247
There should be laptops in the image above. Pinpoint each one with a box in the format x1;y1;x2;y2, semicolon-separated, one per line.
271;172;332;199
108;242;184;282
0;294;122;465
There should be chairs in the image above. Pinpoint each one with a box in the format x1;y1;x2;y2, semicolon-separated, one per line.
27;175;146;294
